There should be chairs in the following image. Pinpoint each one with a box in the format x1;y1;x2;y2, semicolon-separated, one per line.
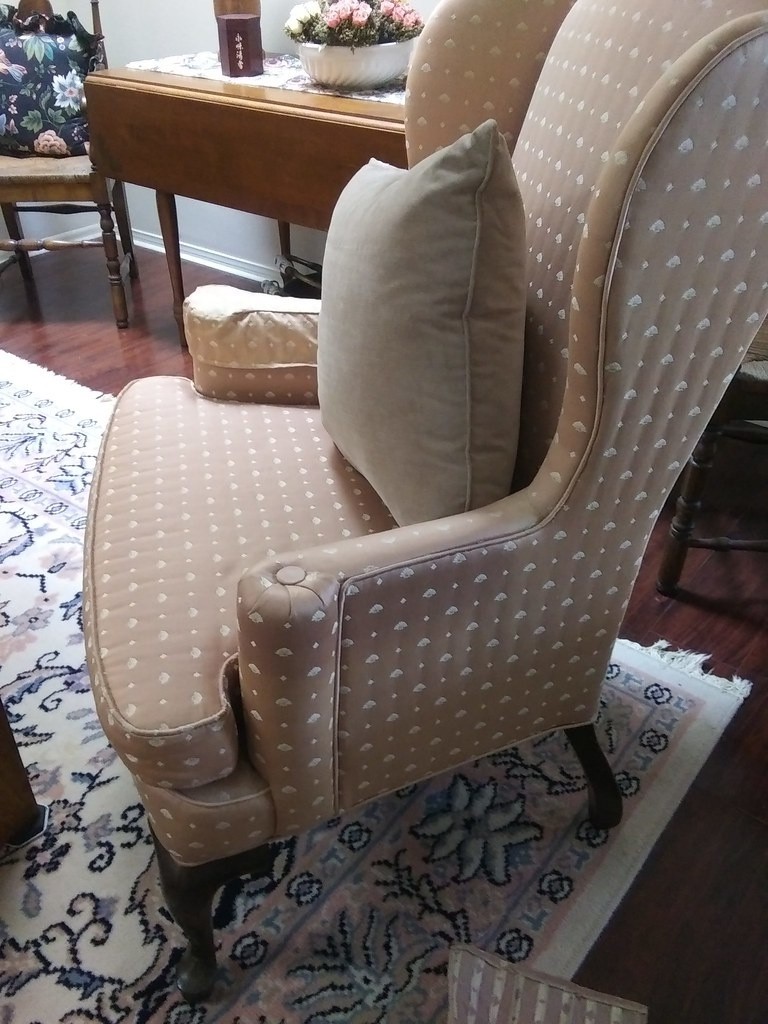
84;0;768;1003
0;0;138;328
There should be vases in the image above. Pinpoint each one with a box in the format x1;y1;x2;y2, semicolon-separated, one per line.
296;36;418;90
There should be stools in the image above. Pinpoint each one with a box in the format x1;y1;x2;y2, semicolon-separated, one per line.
657;316;768;597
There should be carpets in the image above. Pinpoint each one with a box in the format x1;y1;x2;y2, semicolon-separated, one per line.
0;347;750;1024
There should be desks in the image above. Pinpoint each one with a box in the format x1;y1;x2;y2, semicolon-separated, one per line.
83;51;405;345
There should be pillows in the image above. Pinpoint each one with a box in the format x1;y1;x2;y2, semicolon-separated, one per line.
0;3;106;156
318;119;527;525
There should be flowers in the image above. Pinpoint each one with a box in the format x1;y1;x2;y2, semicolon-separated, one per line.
283;0;425;54
